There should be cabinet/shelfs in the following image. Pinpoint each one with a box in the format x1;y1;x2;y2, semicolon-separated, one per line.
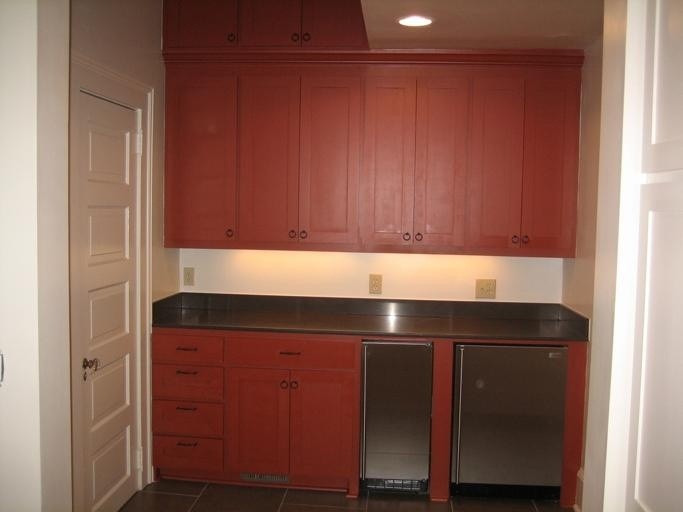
237;0;366;51
360;60;468;250
468;62;574;254
236;61;361;247
451;341;570;487
150;324;225;487
361;340;432;480
224;329;361;495
160;0;236;248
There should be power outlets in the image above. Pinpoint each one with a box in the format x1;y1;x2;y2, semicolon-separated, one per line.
369;274;381;294
